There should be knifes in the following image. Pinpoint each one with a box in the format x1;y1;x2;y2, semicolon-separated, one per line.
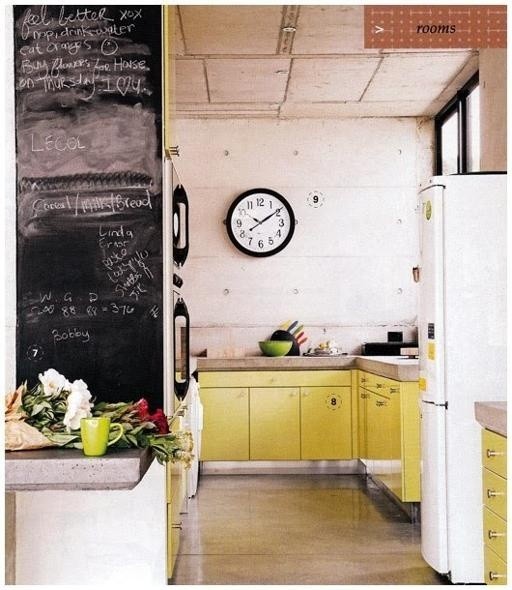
283;319;309;347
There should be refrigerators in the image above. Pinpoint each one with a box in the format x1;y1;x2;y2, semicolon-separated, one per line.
416;173;507;582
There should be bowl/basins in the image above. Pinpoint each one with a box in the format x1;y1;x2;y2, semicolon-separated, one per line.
259;341;293;358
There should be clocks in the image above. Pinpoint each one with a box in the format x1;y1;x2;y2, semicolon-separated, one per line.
226;188;295;258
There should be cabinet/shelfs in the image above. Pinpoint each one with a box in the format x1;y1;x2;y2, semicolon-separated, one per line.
198;369;421;502
163;6;181;160
481;426;507;585
167;415;184;578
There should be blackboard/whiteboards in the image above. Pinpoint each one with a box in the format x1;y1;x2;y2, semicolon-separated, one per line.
14;6;162;415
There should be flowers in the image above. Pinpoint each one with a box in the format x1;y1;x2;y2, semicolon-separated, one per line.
4;367;196;469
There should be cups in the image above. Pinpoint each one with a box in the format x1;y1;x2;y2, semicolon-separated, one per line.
80;417;124;457
